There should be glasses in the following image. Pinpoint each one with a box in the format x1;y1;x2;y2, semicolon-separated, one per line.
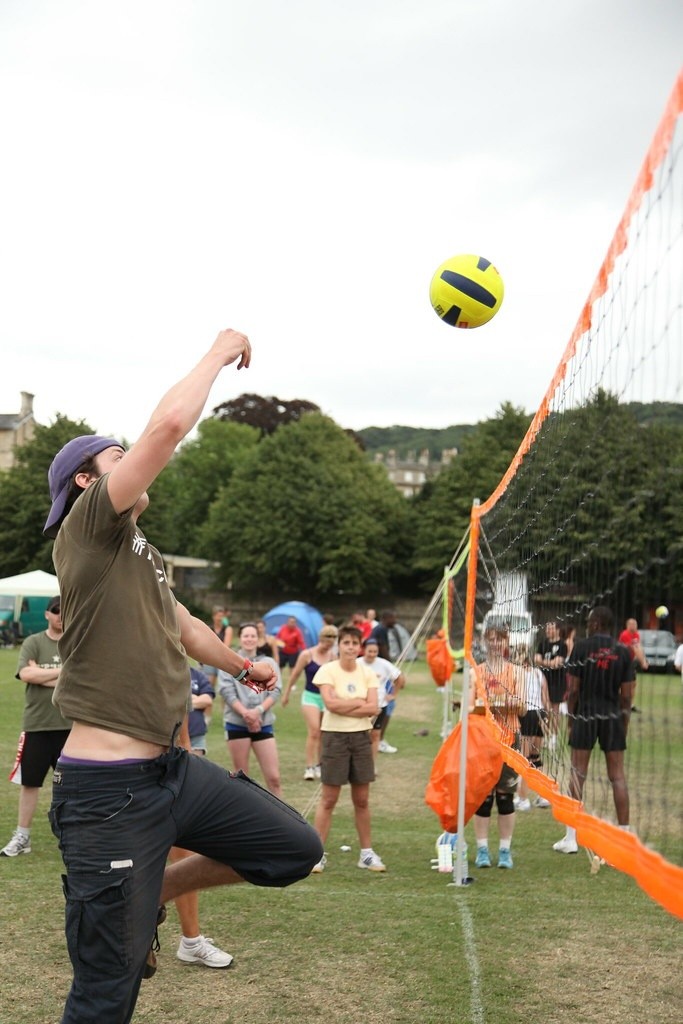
49;607;61;615
323;635;338;639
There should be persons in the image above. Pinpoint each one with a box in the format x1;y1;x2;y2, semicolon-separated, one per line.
168;593;648;967
0;596;73;856
463;615;526;870
674;644;683;680
311;627;388;873
219;624;283;799
45;329;323;1024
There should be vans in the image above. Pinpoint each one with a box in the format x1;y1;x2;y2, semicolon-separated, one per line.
0;594;52;645
475;609;539;664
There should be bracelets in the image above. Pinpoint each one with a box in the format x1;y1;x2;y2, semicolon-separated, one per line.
233;658;263;694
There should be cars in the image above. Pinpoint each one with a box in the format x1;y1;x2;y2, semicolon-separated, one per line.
636;629;676;672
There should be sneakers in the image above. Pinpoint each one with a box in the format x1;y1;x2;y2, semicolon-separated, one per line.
176;935;233;969
497;848;514;869
553;836;578;854
357;851;386;871
0;831;32;856
475;846;491;868
312;853;328;873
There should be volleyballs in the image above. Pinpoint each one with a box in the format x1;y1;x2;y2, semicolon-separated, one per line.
655;606;669;619
430;254;504;329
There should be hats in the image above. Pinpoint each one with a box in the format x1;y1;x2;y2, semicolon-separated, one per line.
47;595;60;610
44;434;126;541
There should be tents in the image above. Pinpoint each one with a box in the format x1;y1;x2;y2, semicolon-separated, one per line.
0;569;61;597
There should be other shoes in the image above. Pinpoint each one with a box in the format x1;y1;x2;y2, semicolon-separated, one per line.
376;739;397;754
314;766;321;778
531;795;548;807
302;767;313;780
514;798;529;810
142;905;168;979
